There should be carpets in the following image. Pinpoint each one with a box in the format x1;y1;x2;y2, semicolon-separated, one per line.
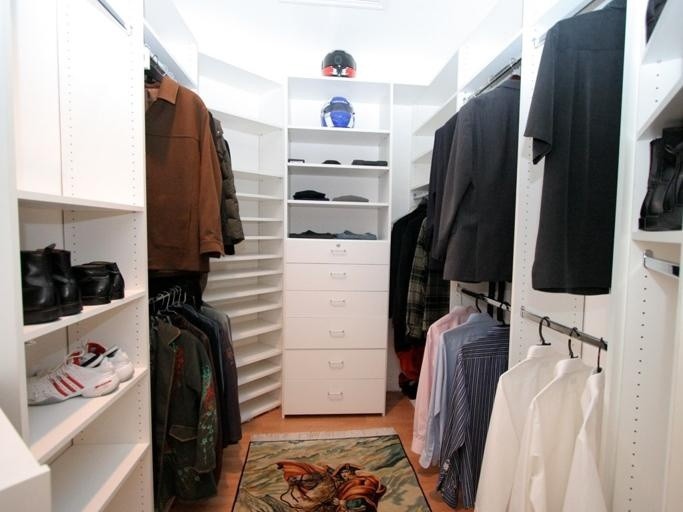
232;427;432;512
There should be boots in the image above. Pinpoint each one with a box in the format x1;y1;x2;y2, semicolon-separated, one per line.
72;259;125;306
20;247;84;326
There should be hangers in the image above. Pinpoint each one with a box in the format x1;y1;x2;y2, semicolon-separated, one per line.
152;287;186;323
475;294;604;372
507;56;521;81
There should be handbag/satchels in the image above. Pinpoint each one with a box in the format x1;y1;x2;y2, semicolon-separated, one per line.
638;126;683;232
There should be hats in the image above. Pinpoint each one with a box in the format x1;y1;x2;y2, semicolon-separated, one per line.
331;463;360;476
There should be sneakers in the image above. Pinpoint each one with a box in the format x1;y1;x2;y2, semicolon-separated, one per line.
26;340;136;406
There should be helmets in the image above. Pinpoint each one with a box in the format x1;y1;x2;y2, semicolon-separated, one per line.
320;96;356;129
321;50;357;79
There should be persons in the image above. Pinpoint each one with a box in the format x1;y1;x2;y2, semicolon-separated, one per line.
329;463;383;511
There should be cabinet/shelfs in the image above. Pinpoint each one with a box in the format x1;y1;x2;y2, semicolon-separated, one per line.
281;77;392;419
161;49;286;425
506;1;683;512
1;1;155;512
405;0;522;213
